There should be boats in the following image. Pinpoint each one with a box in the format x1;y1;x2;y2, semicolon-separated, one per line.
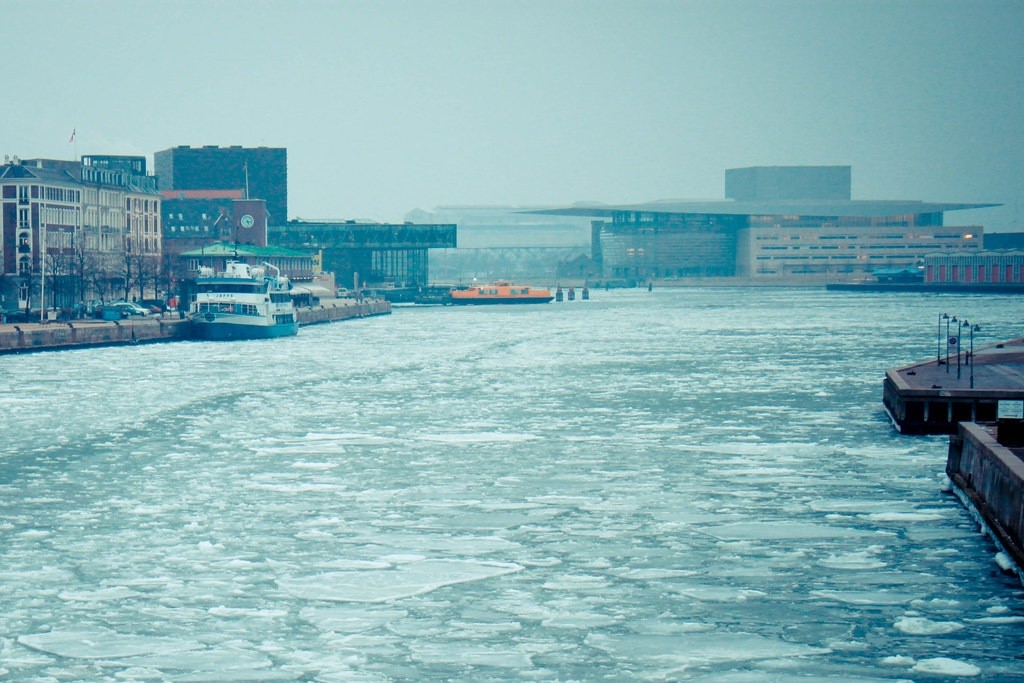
187;250;300;341
451;281;556;305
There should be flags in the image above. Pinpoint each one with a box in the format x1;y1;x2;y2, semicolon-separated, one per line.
69;129;75;142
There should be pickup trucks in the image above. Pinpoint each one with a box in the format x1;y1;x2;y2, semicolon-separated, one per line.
337;288;349;297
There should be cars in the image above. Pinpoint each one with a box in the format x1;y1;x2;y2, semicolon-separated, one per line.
111;303;149;316
143;304;162;313
665;275;677;281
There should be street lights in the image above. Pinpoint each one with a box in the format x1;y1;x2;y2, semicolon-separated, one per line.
947;316;957;373
957;320;969;379
938;313;949;366
971;324;980;388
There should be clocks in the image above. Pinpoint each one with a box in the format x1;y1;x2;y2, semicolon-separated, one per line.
241;215;254;229
